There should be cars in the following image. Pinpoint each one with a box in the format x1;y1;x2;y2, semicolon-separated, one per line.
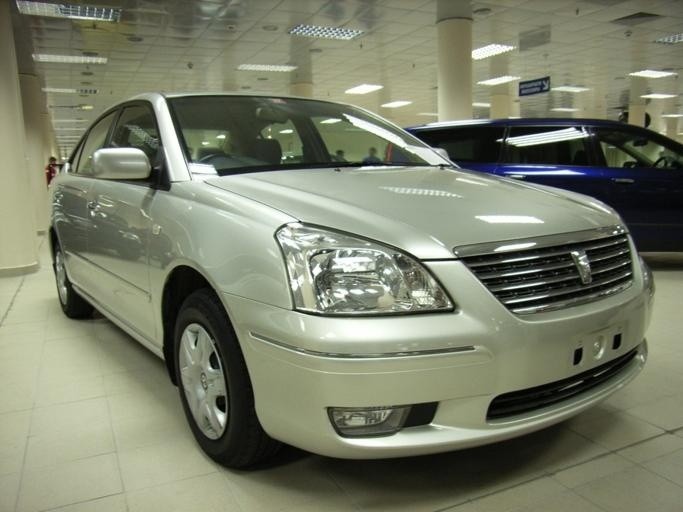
42;93;655;470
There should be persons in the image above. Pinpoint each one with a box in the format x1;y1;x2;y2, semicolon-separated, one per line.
45;157;56;190
362;147;381;163
335;150;347;163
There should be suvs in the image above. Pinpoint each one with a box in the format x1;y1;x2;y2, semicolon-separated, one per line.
382;112;683;274
